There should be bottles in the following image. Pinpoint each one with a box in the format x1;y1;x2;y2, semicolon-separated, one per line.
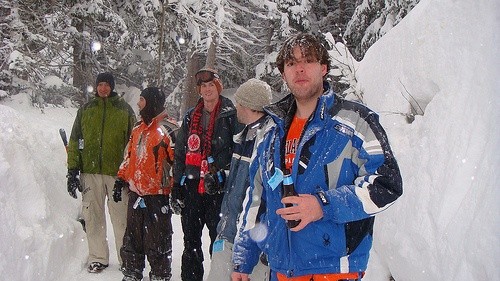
207;154;224;190
282;169;301;228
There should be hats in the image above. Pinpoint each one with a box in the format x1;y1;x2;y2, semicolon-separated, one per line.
96;72;116;92
139;87;166;124
197;68;223;95
234;78;272;110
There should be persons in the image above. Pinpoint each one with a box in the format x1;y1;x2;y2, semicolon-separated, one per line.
170;69;245;281
230;31;403;281
67;71;137;272
112;88;181;281
209;78;273;281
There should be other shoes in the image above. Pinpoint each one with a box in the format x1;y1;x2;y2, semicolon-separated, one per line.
148;269;172;281
122;271;143;281
87;261;106;274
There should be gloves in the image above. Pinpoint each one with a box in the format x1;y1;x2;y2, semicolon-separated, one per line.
66;177;83;199
112;180;124;203
168;189;188;215
205;172;223;194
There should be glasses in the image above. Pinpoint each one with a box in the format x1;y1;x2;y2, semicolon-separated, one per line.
194;70;217;84
